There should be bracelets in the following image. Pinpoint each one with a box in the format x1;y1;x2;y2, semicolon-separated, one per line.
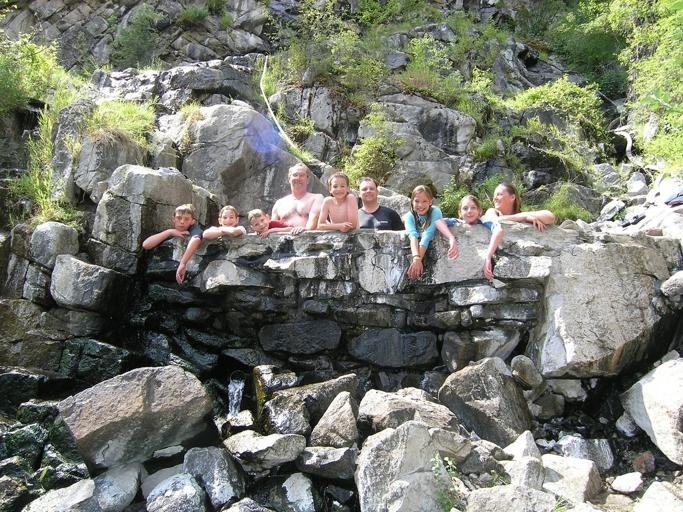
412;258;422;261
412;254;420;258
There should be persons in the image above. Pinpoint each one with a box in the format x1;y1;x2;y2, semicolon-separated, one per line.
356;175;403;231
315;172;357;233
247;208;293;237
403;185;443;279
270;162;324;236
434;194;504;280
141;202;202;285
483;181;555;232
202;205;246;240
